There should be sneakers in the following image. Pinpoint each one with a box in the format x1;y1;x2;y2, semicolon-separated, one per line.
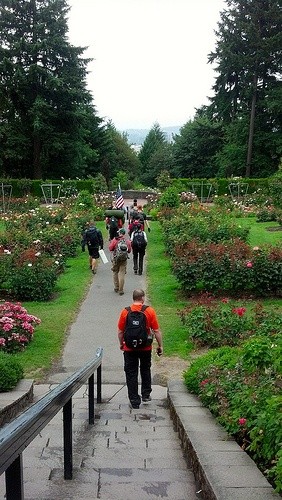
142;397;151;404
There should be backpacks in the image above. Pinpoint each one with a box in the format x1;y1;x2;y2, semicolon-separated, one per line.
129;224;138;237
114;237;128;262
86;227;99;247
104;210;124;218
124;305;153;348
132;231;146;250
138;212;144;221
132;208;139;216
110;216;118;228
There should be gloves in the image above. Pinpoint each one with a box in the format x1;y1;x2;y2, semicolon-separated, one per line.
82;247;85;251
148;228;150;232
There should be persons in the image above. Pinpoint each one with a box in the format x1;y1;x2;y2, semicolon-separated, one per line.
130;223;148;275
81;221;104;274
128;199;138;213
108;228;132;295
133;204;150;232
128;216;144;239
118;288;164;409
106;200;125;241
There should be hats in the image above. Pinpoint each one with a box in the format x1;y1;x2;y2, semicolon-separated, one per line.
117;228;125;235
111;201;116;206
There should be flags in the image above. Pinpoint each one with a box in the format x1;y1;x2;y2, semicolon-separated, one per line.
115;195;124;208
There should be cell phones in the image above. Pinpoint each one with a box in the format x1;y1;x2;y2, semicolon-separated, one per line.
157;348;161;356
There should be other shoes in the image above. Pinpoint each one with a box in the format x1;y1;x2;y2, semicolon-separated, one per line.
90;265;92;269
119;293;124;295
92;270;96;274
134;271;137;273
114;289;118;292
139;272;142;275
